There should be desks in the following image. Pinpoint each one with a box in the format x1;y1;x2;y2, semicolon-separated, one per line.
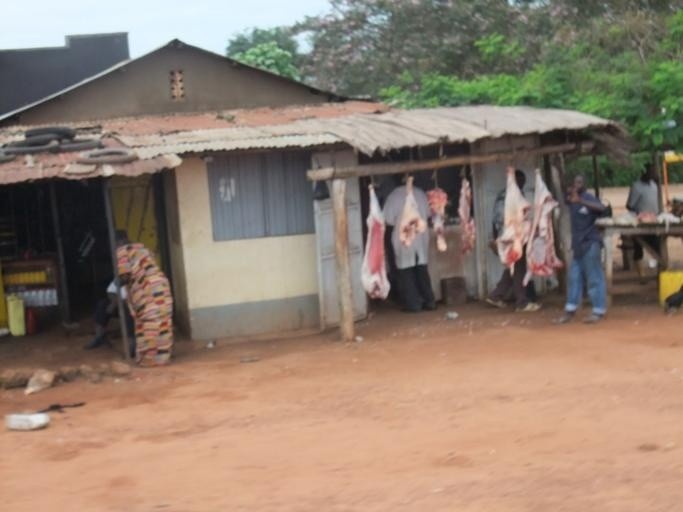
596;219;682;304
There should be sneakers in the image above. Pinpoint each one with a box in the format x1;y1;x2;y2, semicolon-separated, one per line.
558;311;604;324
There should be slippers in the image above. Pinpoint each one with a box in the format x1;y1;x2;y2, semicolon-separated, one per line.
485;297;543;312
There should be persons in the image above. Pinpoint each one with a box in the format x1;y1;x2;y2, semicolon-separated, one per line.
551;174;612;322
381;173;435;312
486;170;543;312
626;160;662;282
106;228;174;365
